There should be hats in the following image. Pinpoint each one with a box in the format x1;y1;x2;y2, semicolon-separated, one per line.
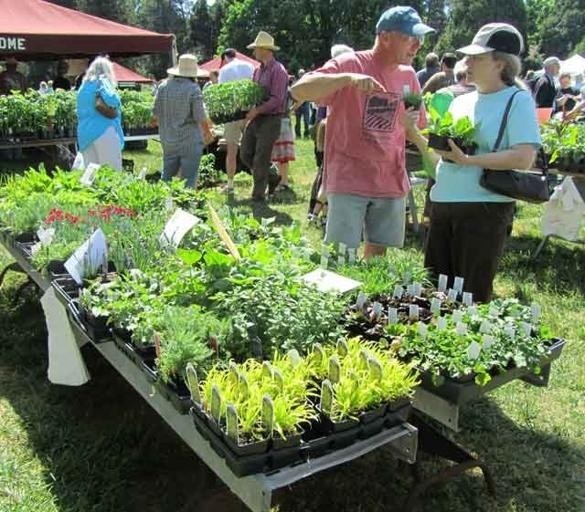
5;57;19;65
247;30;281;52
376;5;437;37
167;54;210;78
455;22;523;56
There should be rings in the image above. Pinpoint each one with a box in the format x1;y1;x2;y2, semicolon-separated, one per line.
466;154;469;159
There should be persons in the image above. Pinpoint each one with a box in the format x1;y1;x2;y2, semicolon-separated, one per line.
203;31;354;227
76;55;125;174
289;4;426;259
0;58;95;169
416;52;585;118
149;55;212;191
399;24;542;303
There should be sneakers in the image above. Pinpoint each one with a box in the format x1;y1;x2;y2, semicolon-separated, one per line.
253;177;268;199
250;173;289;204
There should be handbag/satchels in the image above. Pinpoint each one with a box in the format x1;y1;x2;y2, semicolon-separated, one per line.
479;166;551;205
530;75;555;106
95;97;118;119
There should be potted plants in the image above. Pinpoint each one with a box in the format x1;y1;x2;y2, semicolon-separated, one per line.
1;162;565;511
201;78;266;125
452;118;476;156
0;90;160;143
541;112;585;174
402;88;420;112
427;110;452;149
